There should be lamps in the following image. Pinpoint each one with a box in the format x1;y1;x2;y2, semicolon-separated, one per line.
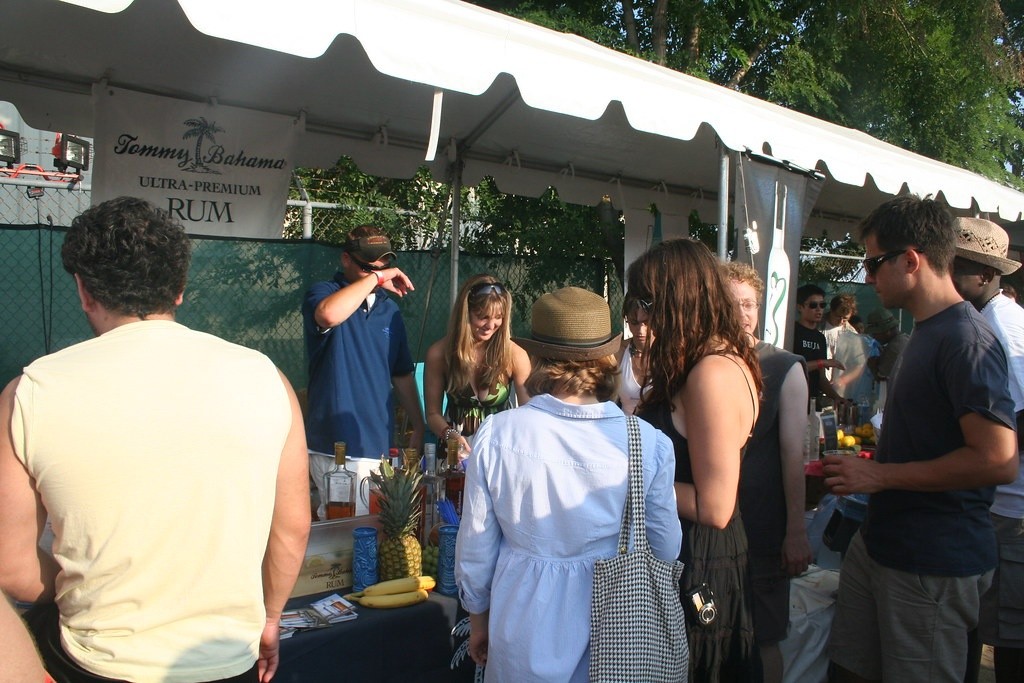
53;133;91;181
0;126;20;168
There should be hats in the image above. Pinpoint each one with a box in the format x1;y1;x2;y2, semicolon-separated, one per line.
863;308;901;333
951;217;1022;276
510;287;623;361
346;225;397;261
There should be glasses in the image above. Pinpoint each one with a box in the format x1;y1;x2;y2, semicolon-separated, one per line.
735;301;762;310
804;302;827;309
471;282;505;297
863;249;924;277
638;299;655;314
347;251;391;272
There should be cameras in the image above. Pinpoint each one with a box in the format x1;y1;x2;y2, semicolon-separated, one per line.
684;583;720;626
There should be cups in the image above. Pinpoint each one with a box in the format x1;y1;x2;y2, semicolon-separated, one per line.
823;450;854;496
360;476;386;515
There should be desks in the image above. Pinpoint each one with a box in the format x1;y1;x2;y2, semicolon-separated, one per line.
262;581;484;683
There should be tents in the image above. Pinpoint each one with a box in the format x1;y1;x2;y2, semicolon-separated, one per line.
0;0;1024;683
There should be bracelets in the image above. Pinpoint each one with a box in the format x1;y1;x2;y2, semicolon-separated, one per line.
440;426;460;443
370;269;384;287
816;359;824;369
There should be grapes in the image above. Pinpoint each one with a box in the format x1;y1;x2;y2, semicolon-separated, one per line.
421;545;439;579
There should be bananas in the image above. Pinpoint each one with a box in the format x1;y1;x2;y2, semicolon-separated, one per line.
342;574;437;609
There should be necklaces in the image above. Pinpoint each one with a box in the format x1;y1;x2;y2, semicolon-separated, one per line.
629;338;651;371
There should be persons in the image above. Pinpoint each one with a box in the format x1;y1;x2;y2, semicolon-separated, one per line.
0;195;312;683
0;586;57;682
719;260;810;683
817;294;914;403
453;286;683;683
822;195;1019;683
614;237;763;683
949;216;1024;683
423;273;535;465
794;283;846;411
302;225;425;521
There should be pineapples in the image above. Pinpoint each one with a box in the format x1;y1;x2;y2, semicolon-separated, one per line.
359;463;423;583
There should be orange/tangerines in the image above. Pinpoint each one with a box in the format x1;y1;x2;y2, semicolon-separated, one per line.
836;422;875;448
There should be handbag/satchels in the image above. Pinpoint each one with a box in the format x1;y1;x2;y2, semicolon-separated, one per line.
589;552;689;683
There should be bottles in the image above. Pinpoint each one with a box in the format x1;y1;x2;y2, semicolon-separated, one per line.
402;449;416;470
419;444;445;549
323;442;357;521
445;439;465;520
389;448;399;469
839;376;887;438
808;397;820;461
803;416;810;465
